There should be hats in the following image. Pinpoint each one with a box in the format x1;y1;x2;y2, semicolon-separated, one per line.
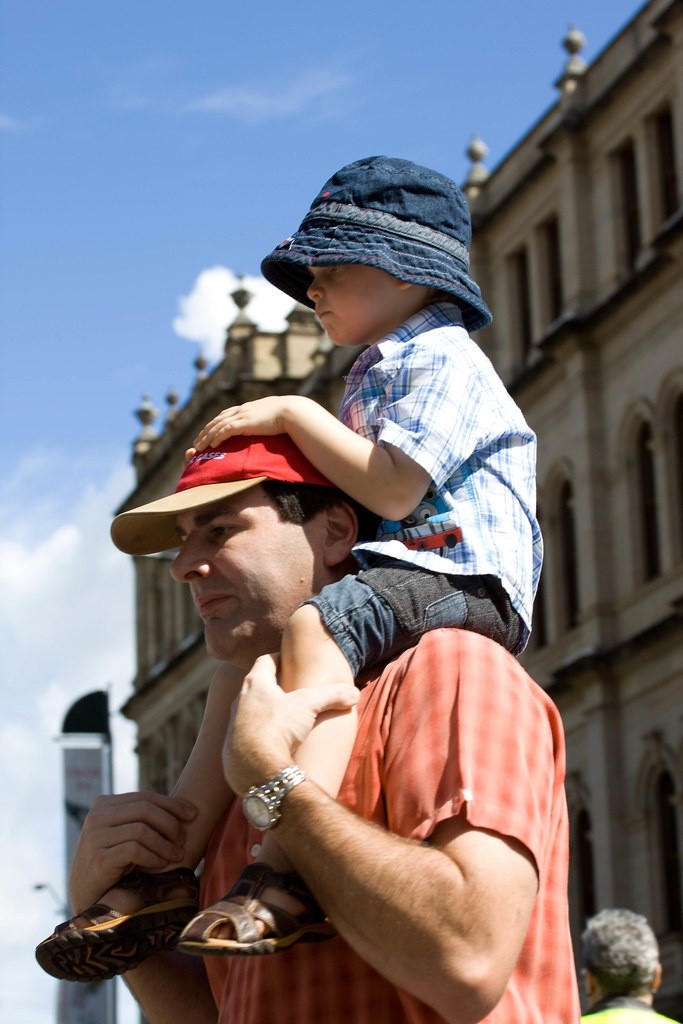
110;433;337;557
261;156;493;334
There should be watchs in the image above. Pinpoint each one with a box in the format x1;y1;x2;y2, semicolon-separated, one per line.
240;764;311;832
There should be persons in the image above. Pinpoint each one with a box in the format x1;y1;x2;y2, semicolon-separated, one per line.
580;907;683;1022
38;152;582;1024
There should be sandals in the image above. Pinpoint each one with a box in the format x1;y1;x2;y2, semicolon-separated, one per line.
178;862;338;958
36;867;200;983
